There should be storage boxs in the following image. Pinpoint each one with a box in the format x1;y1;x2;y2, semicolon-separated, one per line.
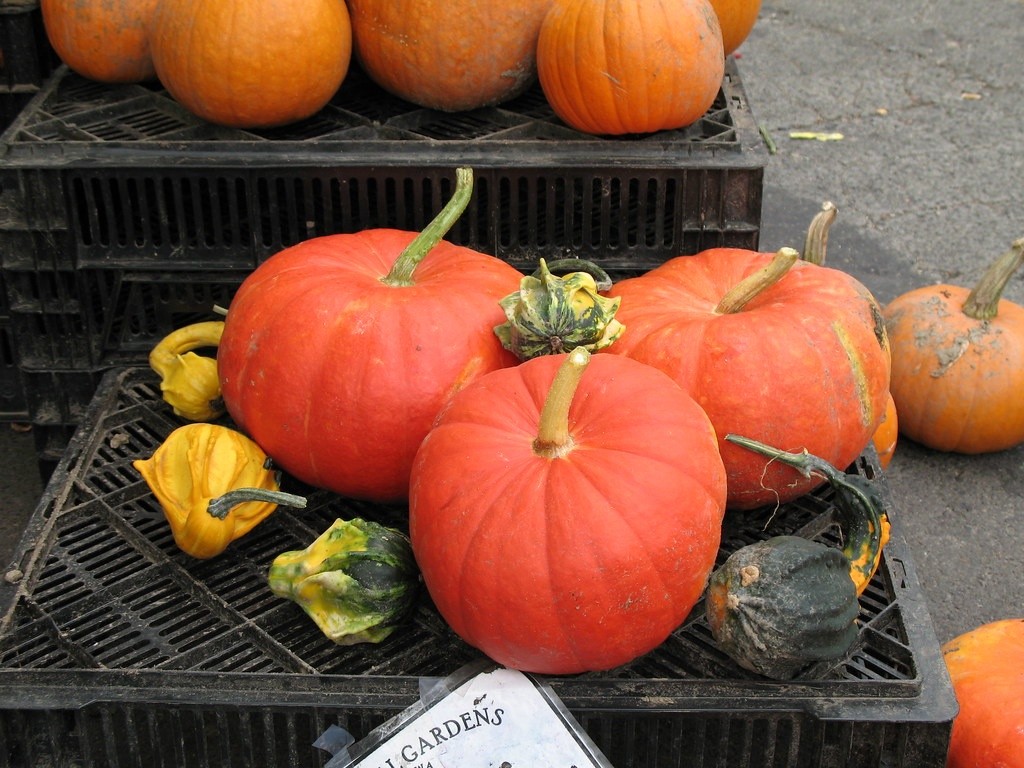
0;366;958;768
0;1;772;488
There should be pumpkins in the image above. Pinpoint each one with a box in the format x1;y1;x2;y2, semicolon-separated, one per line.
937;617;1024;768
882;236;1024;455
134;165;899;683
35;0;762;136
